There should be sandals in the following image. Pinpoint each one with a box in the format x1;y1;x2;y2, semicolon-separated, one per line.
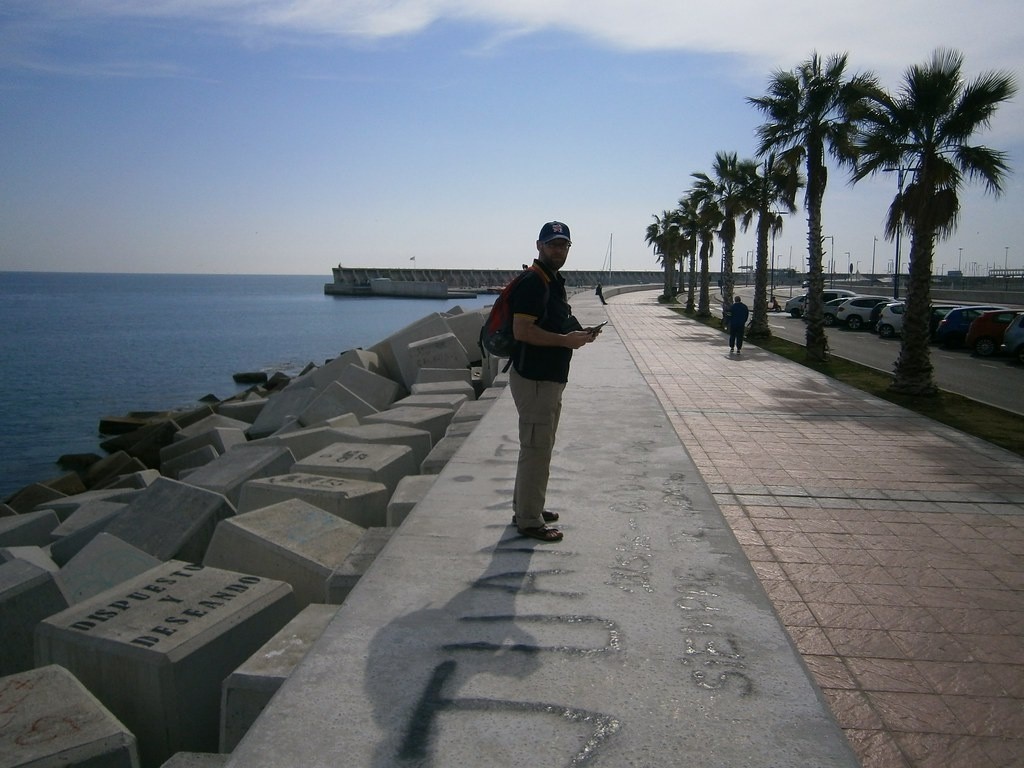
512;509;560;523
517;525;565;542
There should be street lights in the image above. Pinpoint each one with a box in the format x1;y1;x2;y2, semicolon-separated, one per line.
882;165;915;298
845;252;850;281
871;236;878;286
769;210;788;302
825;236;833;289
746;251;752;287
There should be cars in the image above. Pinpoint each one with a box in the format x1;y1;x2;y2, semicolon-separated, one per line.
785;294;806;318
1000;313;1024;365
930;304;981;344
823;298;848;326
965;309;1024;357
876;302;906;338
837;296;899;330
868;302;903;333
936;306;1009;350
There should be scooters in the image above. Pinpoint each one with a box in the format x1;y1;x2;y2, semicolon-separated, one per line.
766;297;781;312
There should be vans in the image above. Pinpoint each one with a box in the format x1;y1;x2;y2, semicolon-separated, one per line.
800;289;857;319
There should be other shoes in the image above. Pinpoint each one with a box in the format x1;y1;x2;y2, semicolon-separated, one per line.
737;350;741;355
603;303;608;305
730;349;735;352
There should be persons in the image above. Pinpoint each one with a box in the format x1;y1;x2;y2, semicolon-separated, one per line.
596;283;607;305
725;296;748;354
510;221;604;540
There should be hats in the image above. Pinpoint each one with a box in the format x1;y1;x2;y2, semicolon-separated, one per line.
538;221;572;242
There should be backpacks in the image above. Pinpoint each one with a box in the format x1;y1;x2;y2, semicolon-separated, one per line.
477;264;535;373
722;305;736;322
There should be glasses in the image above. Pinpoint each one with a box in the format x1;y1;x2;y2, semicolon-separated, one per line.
543;241;572;251
